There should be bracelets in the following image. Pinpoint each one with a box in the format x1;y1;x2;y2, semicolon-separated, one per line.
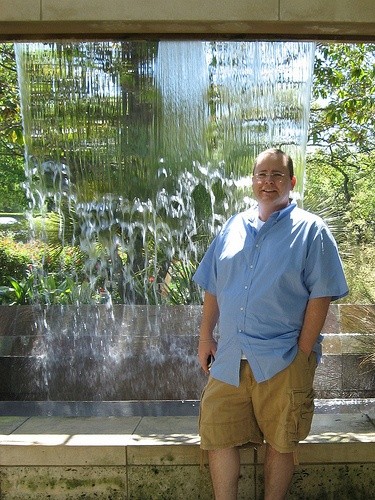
198;338;214;343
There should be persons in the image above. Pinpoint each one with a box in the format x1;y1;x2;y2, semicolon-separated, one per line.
192;148;349;500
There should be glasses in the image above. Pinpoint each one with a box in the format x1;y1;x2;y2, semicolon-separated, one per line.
254;172;291;182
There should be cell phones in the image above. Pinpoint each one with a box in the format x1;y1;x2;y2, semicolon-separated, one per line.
207;354;213;368
